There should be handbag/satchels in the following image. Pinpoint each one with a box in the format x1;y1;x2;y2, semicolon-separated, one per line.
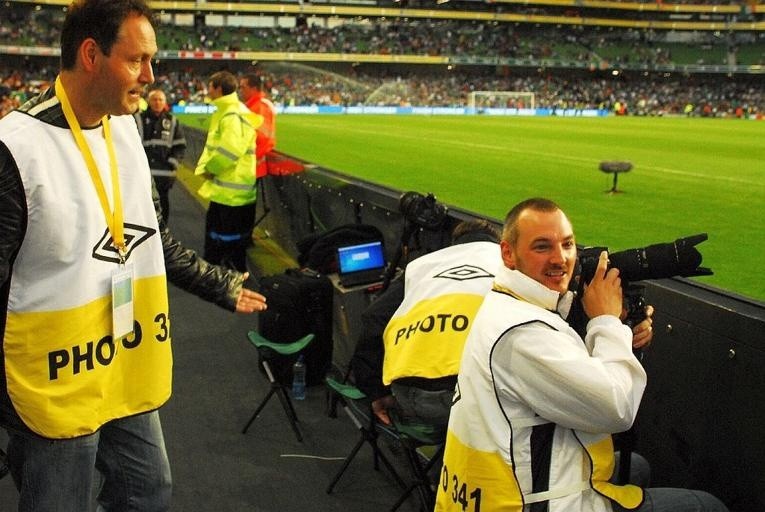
296;219;386;275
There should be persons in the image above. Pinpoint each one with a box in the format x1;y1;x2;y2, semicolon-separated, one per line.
355;218;514;426
133;90;187;225
236;73;275;250
193;71;264;289
0;0;268;510
431;196;730;512
1;0;764;124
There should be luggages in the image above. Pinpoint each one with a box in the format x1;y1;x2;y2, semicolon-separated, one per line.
258;261;335;390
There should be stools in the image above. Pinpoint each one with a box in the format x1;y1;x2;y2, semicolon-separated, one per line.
242;332;445;510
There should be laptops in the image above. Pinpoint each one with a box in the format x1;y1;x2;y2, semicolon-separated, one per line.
335;240;387;289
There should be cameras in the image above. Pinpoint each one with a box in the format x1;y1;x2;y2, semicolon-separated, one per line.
398;191;448;229
576;233;713;300
